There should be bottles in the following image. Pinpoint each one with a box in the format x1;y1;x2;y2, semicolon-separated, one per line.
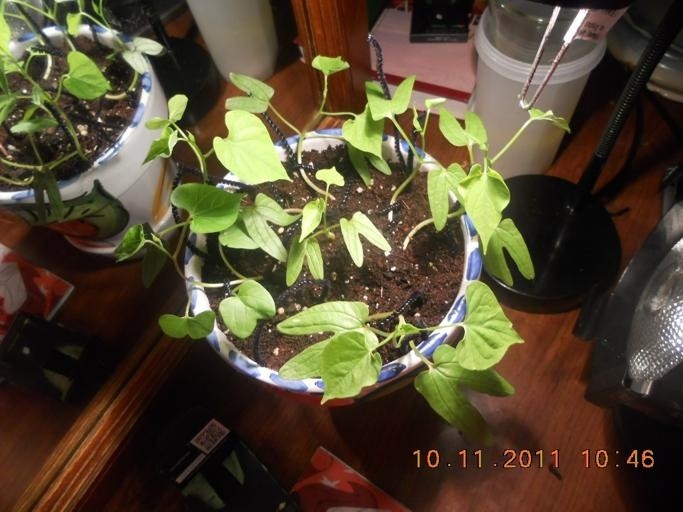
471;0;608;182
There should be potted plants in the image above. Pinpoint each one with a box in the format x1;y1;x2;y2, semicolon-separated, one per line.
1;0;183;261
112;55;572;447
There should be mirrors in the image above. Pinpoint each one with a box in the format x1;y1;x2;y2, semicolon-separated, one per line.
0;0;369;511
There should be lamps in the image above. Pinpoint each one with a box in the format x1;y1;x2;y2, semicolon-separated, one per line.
574;201;683;448
